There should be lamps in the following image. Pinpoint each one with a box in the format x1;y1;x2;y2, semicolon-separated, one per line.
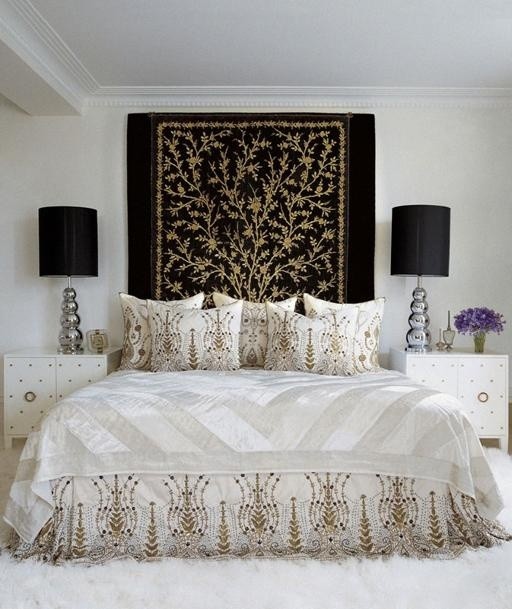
391;205;451;355
38;205;97;355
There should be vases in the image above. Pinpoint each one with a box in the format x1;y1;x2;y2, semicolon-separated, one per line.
472;327;485;354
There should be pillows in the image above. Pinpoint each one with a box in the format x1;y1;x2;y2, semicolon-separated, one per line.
210;289;298;368
144;296;246;374
302;290;388;377
261;296;362;379
117;287;206;370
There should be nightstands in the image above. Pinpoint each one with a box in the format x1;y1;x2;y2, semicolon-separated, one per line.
3;346;125;452
387;344;510;457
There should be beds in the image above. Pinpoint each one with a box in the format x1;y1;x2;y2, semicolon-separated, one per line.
1;364;511;566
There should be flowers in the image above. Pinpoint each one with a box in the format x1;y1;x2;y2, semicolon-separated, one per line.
451;307;505;348
